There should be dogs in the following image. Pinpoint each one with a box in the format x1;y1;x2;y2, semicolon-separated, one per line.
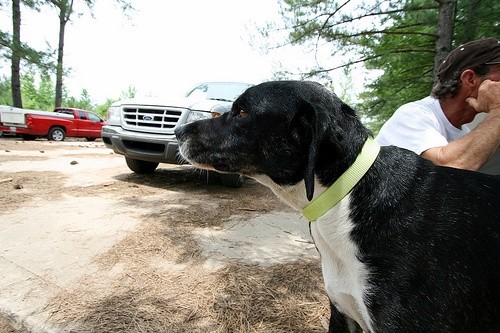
174;80;500;333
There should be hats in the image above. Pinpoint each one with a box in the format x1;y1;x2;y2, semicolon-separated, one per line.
436;37;499;79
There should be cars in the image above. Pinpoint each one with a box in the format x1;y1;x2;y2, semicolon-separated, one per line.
101;81;257;187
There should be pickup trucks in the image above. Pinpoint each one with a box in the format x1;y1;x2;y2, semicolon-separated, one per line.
0;107;108;141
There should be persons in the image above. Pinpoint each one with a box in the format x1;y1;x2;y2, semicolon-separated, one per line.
375;38;500;175
